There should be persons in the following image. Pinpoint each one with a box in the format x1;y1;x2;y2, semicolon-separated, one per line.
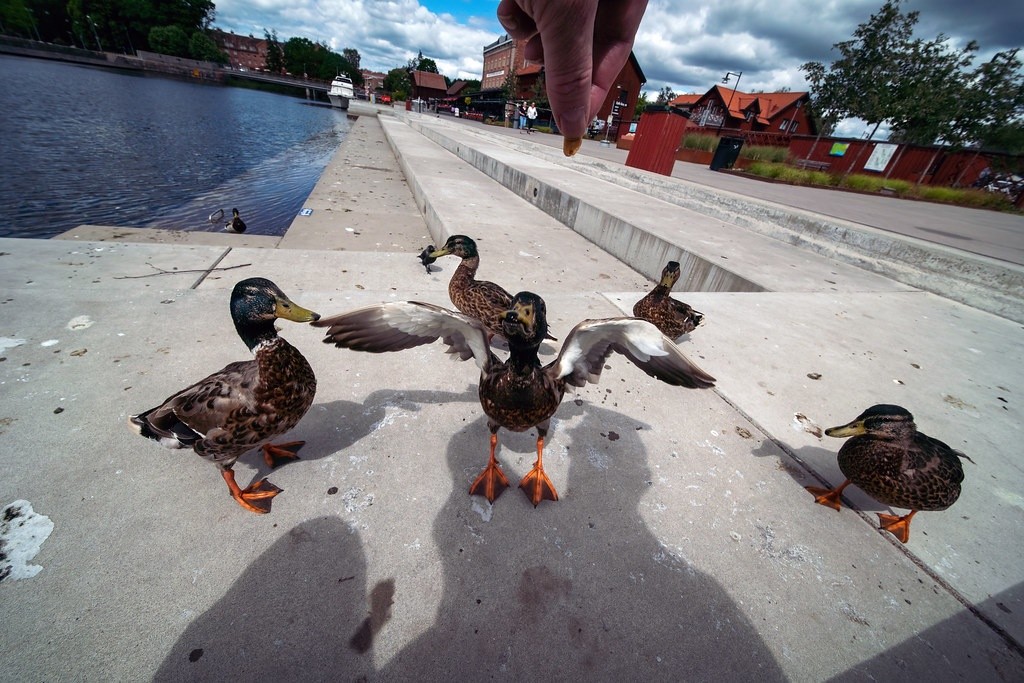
526;101;538;135
518;101;528;135
496;0;650;143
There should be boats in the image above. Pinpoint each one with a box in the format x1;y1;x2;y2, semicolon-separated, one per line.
327;74;355;108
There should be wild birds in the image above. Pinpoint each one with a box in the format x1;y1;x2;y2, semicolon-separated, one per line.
419;244;438;274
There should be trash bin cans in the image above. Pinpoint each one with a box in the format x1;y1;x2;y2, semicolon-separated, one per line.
625;104;691;176
710;136;745;171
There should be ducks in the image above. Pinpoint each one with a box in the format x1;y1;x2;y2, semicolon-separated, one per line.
310;291;716;509
633;261;706;342
224;209;247;234
429;235;558;346
130;278;321;514
803;405;974;544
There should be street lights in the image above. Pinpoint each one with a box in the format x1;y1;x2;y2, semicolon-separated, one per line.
600;84;621;148
716;72;742;137
785;99;803;136
860;131;869;139
87;15;103;52
418;51;424;113
25;8;41;41
